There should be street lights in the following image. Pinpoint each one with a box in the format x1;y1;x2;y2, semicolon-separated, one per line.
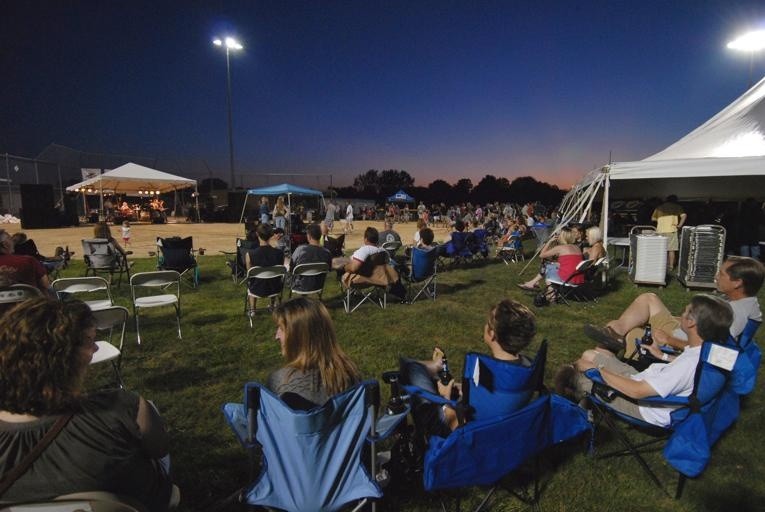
213;36;244;191
726;29;765;90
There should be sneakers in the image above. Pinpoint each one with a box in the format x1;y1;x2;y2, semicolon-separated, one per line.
575;403;594;423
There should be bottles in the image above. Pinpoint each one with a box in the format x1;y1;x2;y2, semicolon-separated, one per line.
387;375;407;432
439;357;450;385
642;323;652;357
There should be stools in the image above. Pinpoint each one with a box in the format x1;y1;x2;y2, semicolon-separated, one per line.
0;284;45;313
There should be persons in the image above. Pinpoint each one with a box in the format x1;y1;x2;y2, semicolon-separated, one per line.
395;228;438;272
267;297;362;412
93;221;133;257
378;220;402;248
414;218;427;243
443;221;466;244
398;299;539;447
343;227;391;273
586;256;765;360
274;196;288;228
246;223;285;317
118;221;132;247
0;296;180;512
325;200;336;232
270;228;284;249
466;199;517;263
517;200;562;223
651;195;685;270
104;196;169;225
290;225;332;273
584;226;606;263
543;228;585;301
517;223;584;289
0;229;49;295
344;200;353;231
557;294;733;427
354;199;466;227
11;233;75;272
257;196;272;223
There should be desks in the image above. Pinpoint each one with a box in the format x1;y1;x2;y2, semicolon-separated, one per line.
607;237;634;271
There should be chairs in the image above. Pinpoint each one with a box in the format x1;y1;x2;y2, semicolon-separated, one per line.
131;270;182;345
157;236;199;289
220;380;410;510
231;238;260;287
583;338;743;502
245;265;286;328
80;238;135;290
560;257;606;303
437;231;472;267
399;245;438;305
288;262;329;303
382;240;402;264
495;230;518;266
653;317;762;409
381;340;594;510
513;233;528;263
342;251;389;315
2;483;182;512
51;277;128;345
541;259;596;305
86;306;130;387
459;229;489;265
15;239;75;283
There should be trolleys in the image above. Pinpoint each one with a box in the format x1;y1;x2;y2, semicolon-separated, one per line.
676;224;727;294
628;225;667;289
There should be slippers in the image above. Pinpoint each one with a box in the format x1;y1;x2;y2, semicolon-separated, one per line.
431;346;446;377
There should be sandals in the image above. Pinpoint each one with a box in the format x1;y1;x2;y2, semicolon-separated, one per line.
584;324;625;351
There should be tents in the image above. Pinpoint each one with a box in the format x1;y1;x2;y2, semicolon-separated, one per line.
386;190;415;210
240;182;325;223
562;78;765;286
65;161;201;225
